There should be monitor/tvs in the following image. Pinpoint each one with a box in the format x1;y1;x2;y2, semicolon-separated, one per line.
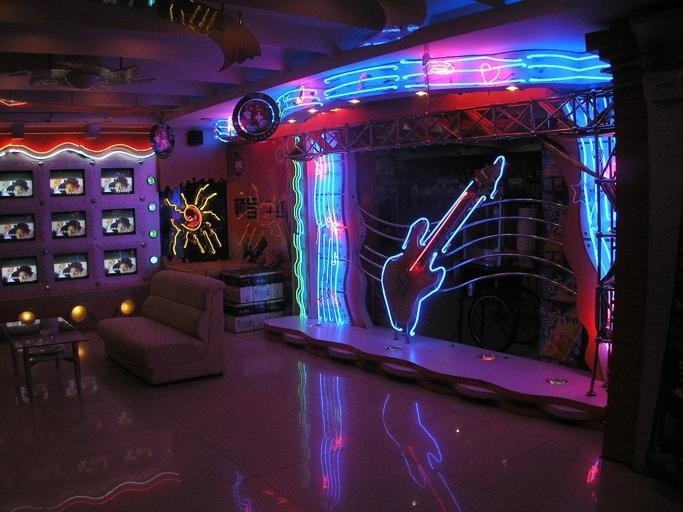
53;252;88;281
0;170;34;199
103;248;137;276
0;213;35;242
51;211;86;239
102;208;136;235
0;256;38;286
101;168;134;195
49;169;85;197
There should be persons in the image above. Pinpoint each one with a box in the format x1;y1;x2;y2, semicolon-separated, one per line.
2;176;133;282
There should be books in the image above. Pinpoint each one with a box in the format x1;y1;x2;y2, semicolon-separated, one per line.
6;317;72;335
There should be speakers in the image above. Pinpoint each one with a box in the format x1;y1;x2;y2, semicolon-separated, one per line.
188;131;203;144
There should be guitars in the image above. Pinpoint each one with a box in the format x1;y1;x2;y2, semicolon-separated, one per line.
379;154;506;336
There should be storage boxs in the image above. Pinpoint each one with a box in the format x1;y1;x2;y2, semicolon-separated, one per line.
218;265;291;334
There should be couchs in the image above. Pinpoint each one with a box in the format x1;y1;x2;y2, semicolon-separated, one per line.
96;268;227;389
